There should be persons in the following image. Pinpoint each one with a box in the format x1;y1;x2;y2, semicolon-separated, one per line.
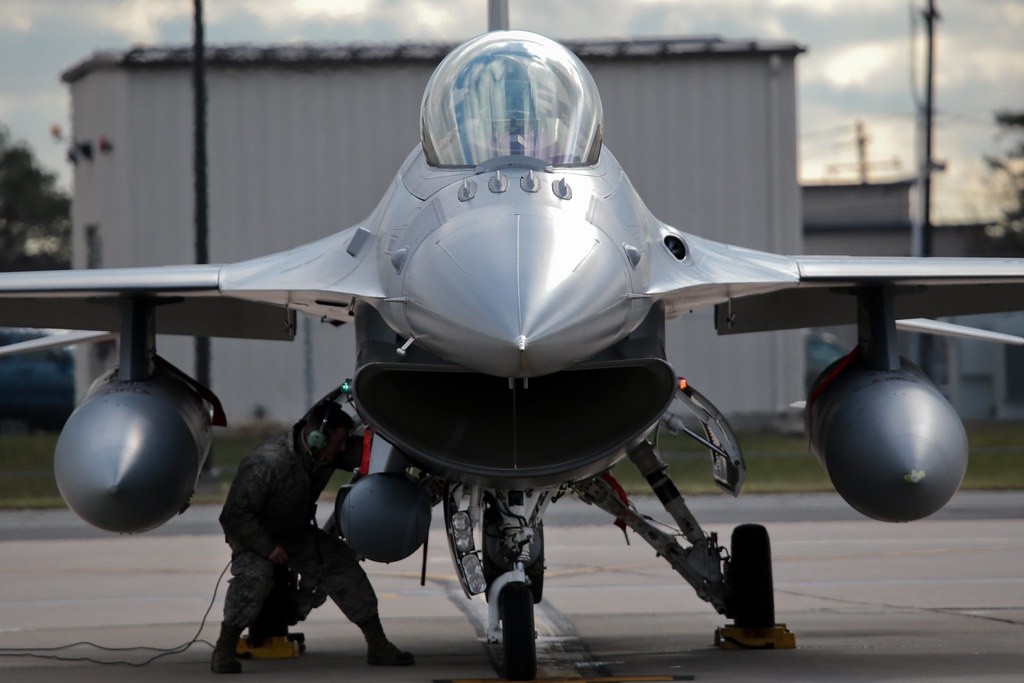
210;412;416;676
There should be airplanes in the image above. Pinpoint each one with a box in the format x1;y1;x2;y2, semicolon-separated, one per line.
0;30;1024;682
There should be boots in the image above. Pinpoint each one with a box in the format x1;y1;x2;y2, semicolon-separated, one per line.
358;613;415;665
211;621;245;674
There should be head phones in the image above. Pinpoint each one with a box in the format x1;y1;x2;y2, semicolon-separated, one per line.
308;402;337;451
